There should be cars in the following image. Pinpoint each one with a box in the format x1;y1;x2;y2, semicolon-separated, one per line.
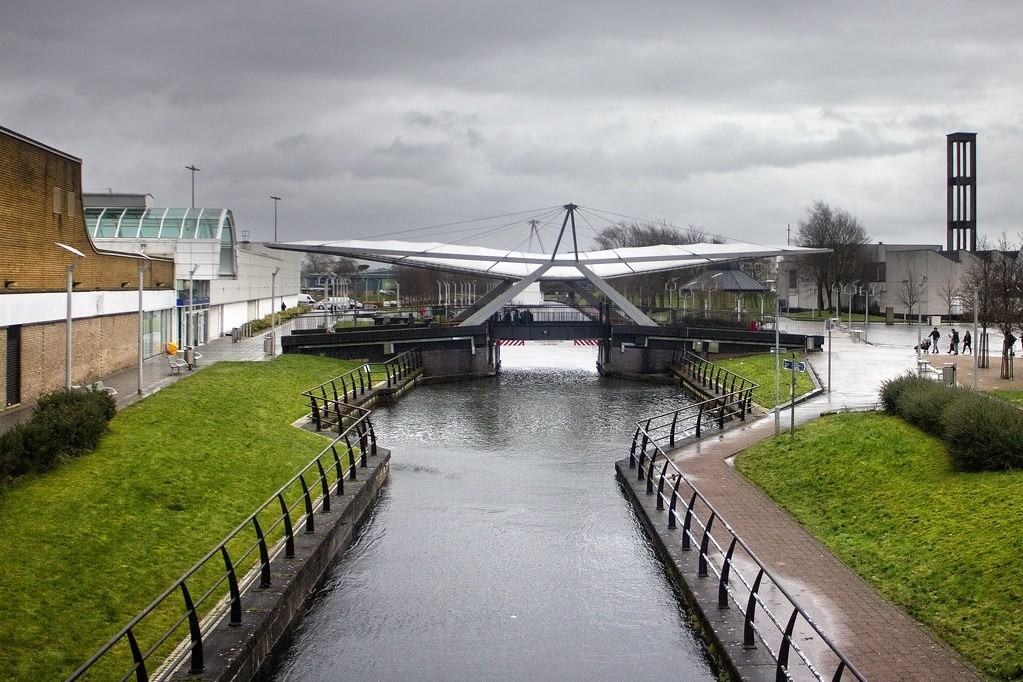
418;306;457;318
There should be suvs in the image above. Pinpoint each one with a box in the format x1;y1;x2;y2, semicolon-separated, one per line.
380;289;395;295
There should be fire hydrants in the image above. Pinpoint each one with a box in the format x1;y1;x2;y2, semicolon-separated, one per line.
747;319;758;334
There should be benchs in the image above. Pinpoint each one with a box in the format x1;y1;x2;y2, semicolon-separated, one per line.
917;359;931;372
851;330;863;341
87;380;117;397
166;354;188;376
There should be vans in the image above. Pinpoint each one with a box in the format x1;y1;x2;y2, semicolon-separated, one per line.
297;294;317;306
314;297;364;311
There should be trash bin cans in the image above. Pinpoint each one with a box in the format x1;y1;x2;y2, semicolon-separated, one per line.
183;346;194;370
263;336;272;354
807;337;814;351
232;328;238;343
943;363;956;384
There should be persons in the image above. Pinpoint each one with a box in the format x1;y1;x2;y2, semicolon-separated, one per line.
520;309;533;324
418;306;425;316
947;328;959;355
1009;334;1015;356
928;327;940;353
503;309;511;325
961;331;972;354
407;313;415;328
281;303;286;312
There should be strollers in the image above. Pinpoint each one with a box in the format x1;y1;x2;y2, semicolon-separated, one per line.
914;336;931;354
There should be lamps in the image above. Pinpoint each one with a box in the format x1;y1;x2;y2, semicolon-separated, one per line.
73;281;83;288
121;280;130;288
156;282;165;288
5;281;17;287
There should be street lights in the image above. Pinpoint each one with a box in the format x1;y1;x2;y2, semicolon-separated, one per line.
774;275;781;436
188;263;202;353
965;276;985;393
271;265;280;359
185;164;200;209
132;246;151;396
58;242;86;396
315;271;352;325
843;286;857;333
831;284;843;318
859;287;875;325
783;352;805;444
270;195;281;243
